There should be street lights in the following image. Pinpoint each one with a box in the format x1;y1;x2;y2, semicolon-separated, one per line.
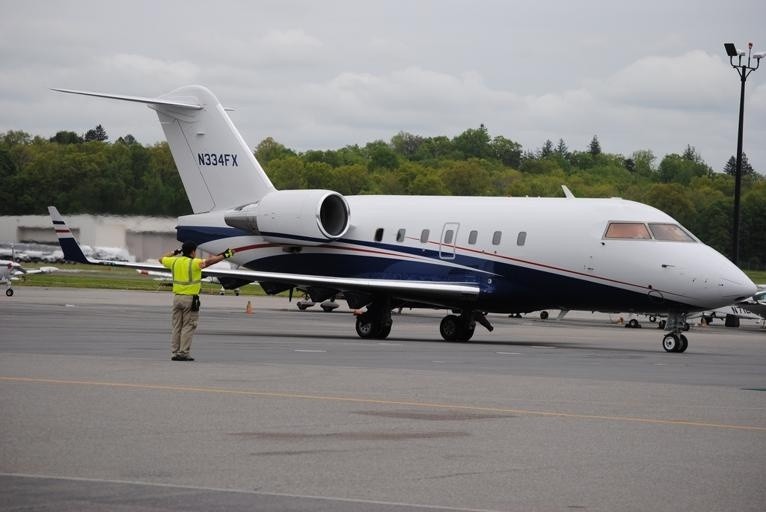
722;40;766;266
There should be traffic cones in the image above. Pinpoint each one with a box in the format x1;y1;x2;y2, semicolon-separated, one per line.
244;300;255;314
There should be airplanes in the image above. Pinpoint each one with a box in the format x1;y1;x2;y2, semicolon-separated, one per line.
48;83;760;354
0;246;38;295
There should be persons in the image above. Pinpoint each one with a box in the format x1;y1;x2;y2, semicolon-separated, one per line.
157;239;236;362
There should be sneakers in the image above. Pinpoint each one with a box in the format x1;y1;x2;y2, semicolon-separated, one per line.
172;353;195;361
